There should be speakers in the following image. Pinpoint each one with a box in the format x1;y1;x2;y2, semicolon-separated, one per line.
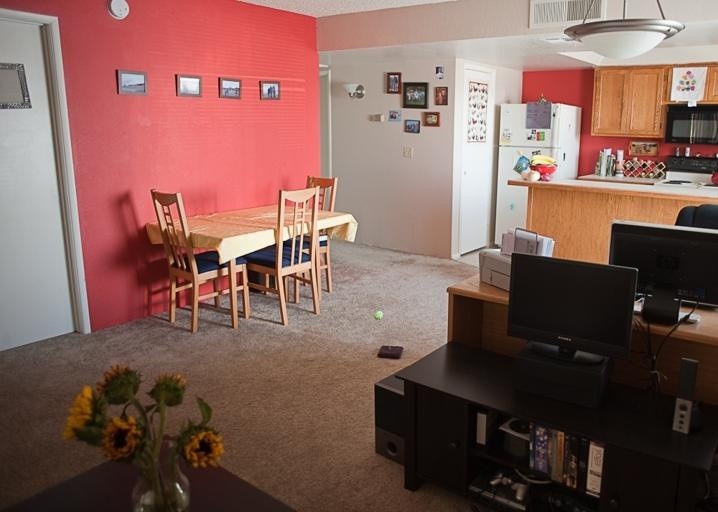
375;369;412;468
672;357;702;437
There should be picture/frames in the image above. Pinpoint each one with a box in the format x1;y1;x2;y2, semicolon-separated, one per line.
387;65;448;133
117;68;147;95
260;80;280;100
219;76;242;98
176;74;202;97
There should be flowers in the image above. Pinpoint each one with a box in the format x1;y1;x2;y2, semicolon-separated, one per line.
63;363;225;512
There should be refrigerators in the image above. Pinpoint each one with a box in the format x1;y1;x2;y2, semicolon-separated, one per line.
492;103;584;246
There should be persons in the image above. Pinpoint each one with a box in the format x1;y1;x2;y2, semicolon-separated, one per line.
225;88;228;97
267;85;276;99
383;63;449;132
235;85;238;94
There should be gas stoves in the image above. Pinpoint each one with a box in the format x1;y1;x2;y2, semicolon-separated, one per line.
653;179;717;190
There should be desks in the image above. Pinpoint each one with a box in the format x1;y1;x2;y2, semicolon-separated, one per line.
0;434;297;512
146;205;352;329
447;274;718;407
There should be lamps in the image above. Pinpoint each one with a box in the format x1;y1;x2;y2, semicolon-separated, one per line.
342;83;364;99
564;0;685;60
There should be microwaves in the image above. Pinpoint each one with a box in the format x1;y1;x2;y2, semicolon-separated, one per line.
665;103;717;144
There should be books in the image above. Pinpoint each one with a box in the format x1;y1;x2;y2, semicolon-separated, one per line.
528;425;604;501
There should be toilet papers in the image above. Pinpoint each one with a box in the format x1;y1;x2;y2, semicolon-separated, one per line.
615;150;624;175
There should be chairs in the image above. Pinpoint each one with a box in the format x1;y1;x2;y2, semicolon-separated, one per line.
150;189;250;334
265;176;337;294
242;186;320;326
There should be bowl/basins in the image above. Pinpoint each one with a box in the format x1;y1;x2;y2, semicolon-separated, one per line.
529;163;558;177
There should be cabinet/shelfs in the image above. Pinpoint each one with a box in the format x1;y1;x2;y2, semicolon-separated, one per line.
666;62;718;104
591;63;668;139
394;342;718;512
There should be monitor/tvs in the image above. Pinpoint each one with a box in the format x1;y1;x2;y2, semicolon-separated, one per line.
607;223;718;325
507;253;638;366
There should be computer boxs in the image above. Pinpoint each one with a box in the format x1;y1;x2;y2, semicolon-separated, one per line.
514;346;612;410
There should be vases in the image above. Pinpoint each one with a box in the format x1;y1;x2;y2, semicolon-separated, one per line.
130;447;191;512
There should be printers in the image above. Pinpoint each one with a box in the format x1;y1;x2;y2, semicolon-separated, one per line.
479;228;556;295
